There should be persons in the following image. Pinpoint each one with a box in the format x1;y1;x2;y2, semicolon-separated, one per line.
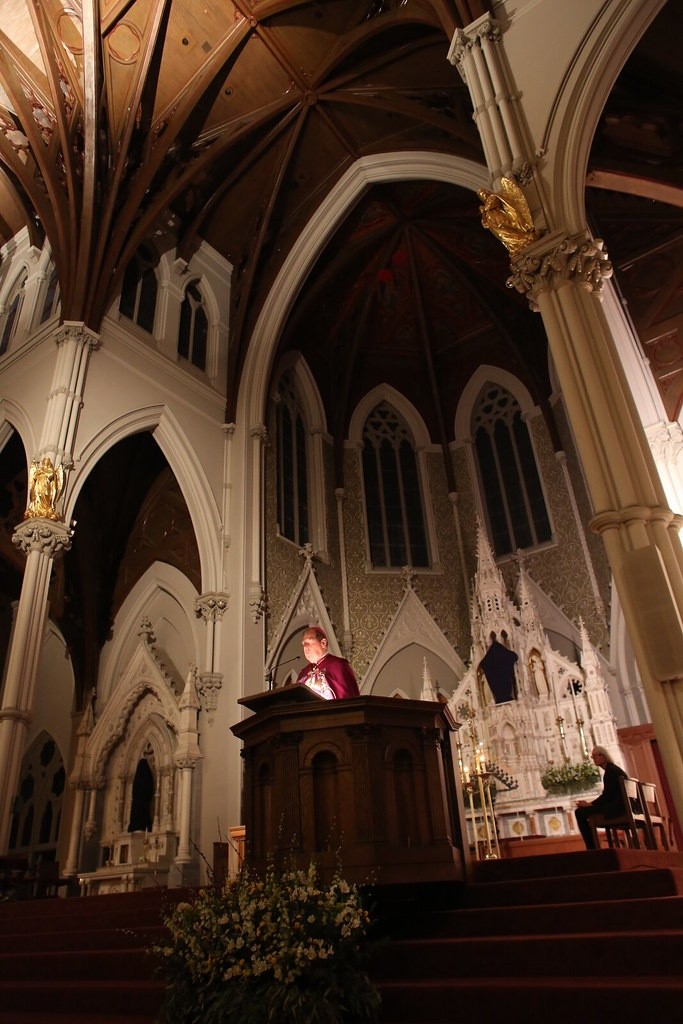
575;746;636;851
25;455;63;522
532;656;549;695
478;177;539;257
296;626;361;700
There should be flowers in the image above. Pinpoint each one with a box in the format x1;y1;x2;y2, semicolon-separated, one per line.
114;814;384;986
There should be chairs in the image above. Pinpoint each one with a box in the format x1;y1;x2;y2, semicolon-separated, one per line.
588;776;655;850
613;782;671;851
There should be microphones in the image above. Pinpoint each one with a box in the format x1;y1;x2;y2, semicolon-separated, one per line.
268;656;300;690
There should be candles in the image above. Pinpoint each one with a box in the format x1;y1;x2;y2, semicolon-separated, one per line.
465;773;471;783
481;762;487;773
